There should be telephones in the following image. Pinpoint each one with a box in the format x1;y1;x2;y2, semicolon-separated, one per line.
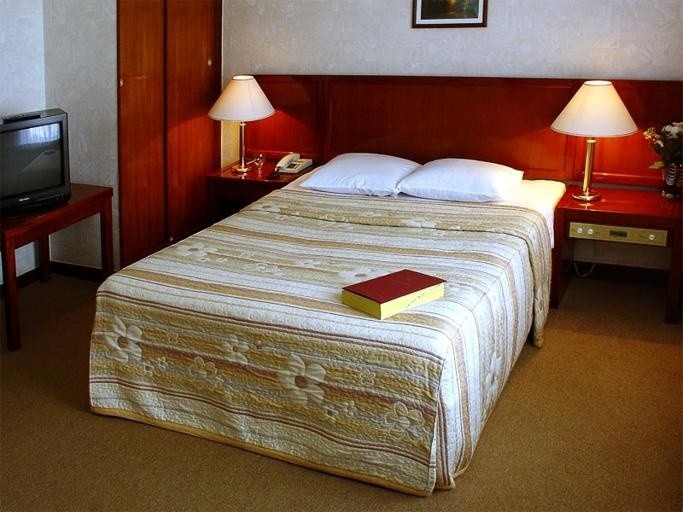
275;153;313;173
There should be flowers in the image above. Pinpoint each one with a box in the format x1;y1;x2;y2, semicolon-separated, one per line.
643;121;683;170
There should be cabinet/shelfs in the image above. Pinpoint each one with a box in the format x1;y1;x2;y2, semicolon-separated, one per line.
42;0;222;269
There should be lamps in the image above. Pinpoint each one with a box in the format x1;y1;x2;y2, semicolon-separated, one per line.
548;78;638;201
208;74;276;172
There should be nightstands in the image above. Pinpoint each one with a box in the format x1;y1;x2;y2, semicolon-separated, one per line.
552;184;683;324
204;157;319;227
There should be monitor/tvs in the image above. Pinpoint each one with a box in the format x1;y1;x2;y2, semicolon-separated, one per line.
0;107;73;225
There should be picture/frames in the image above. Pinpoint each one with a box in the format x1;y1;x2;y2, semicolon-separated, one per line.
412;0;489;28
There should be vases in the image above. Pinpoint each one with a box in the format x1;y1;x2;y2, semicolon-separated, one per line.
662;162;683;201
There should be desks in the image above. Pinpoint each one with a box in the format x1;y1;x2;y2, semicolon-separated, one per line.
0;183;113;352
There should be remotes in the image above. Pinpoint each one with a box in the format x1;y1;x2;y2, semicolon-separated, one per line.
2;112;40;123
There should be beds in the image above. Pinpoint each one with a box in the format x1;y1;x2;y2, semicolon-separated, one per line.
88;160;566;497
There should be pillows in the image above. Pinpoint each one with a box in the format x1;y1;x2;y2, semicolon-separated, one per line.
396;157;525;203
299;151;423;196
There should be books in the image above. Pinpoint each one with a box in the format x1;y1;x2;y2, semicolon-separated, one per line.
341;268;447;321
